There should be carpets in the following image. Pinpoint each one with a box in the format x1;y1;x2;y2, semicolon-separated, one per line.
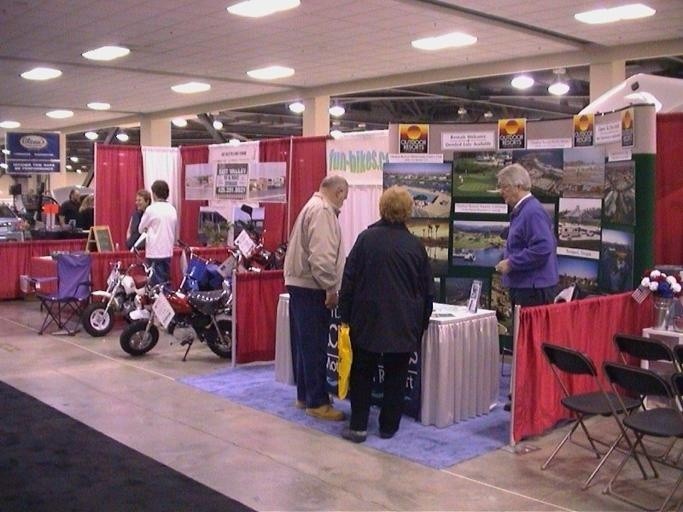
176;363;512;468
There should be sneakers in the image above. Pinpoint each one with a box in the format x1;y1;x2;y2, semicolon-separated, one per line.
342;427;366;443
297;394;346;420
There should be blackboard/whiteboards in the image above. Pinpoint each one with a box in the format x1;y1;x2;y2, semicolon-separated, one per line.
93;225;116;253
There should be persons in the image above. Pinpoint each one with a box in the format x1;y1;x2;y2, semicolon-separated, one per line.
60;189;95;230
338;184;435;442
126;190;151;250
494;163;560;411
282;174;349;422
137;180;178;294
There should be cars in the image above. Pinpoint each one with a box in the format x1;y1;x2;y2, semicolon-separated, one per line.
0;204;26;241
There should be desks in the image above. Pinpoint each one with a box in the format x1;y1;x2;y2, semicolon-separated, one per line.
29;256;59;300
274;293;500;429
641;328;683;412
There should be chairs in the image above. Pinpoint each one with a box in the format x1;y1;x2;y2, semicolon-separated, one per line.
541;334;683;512
25;252;93;336
499;281;580;377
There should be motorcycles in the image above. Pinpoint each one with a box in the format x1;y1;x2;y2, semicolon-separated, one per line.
120;259;231;361
217;204;287;278
170;238;221;298
80;243;168;336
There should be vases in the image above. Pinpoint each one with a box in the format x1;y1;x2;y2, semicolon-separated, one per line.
654;296;679;331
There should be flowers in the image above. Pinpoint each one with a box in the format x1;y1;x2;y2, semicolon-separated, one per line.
641;269;683;297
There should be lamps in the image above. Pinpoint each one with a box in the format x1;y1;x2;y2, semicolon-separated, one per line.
331;126;345;140
511;73;534;90
213;119;223;130
116;128;129;142
329;99;345;117
548;68;570;95
289;102;305;113
172;119;188;128
85;130;99;140
